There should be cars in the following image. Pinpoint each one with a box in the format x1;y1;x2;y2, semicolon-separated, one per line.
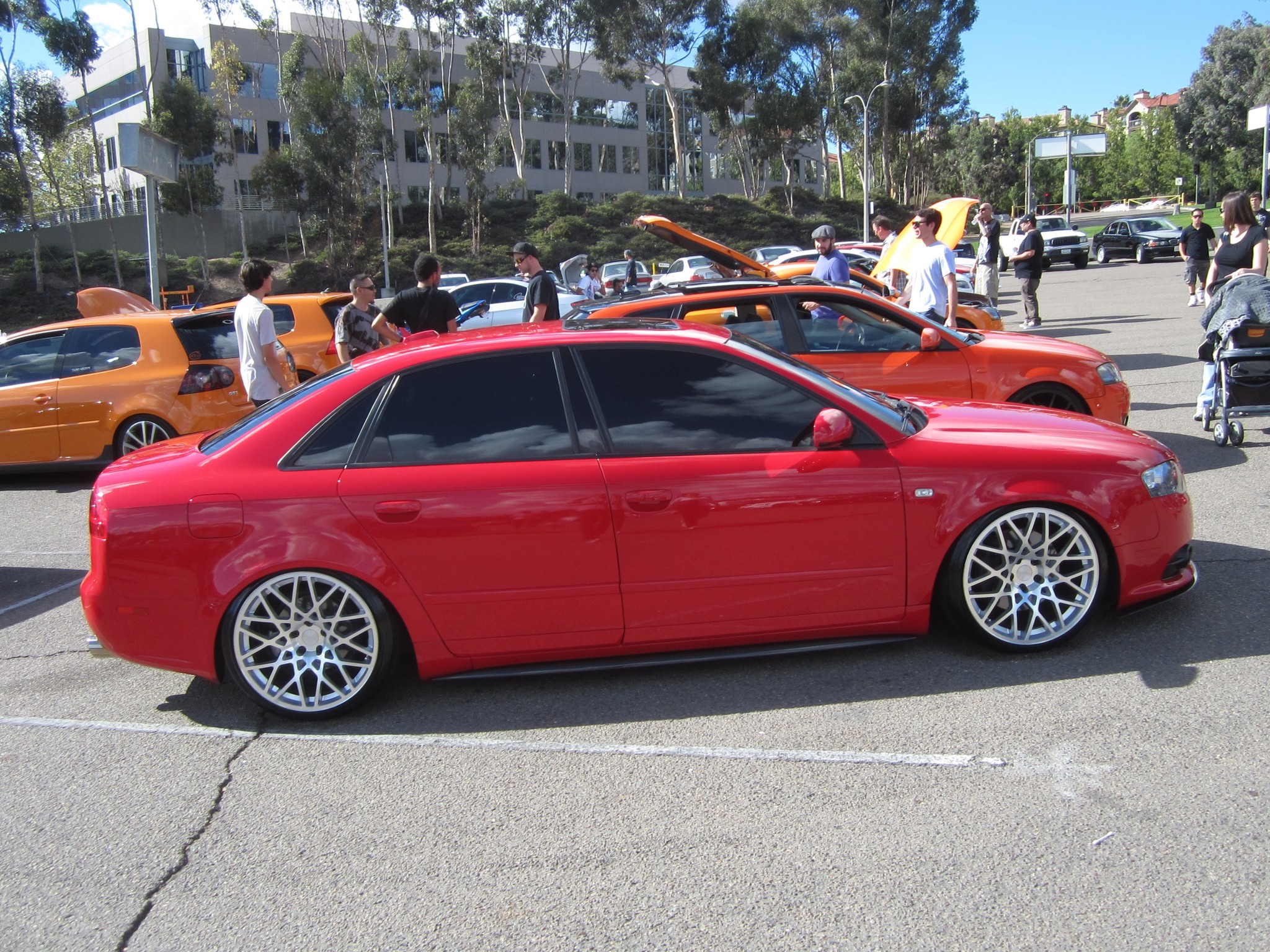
1100;204;1125;213
77;287;398;383
79;316;1198;725
0;307;300;467
437;273;469;292
588;275;1130;428
1091;215;1182;264
448;253;592;331
559;197;1005;332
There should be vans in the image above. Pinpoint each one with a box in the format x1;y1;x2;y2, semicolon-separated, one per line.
1136;199;1166;210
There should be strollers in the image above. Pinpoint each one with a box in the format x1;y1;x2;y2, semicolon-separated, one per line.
1201;276;1270;447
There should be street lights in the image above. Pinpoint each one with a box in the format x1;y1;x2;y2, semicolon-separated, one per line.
843;79;894;245
1027;130;1058;214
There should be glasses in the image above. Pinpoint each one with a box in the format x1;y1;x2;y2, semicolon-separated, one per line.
590;269;599;273
912;222;926;228
978;208;989;211
615;286;622;288
1193;215;1202;218
514;254;529;263
1220;209;1225;213
355;285;375;291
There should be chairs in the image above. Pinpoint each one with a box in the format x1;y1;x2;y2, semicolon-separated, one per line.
724;314;765;336
1144;225;1155;232
1042;225;1051;230
796;309;813;334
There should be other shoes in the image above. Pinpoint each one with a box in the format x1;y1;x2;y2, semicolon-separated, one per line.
1193;406;1218;420
1019;321;1028;327
1023;323;1041;329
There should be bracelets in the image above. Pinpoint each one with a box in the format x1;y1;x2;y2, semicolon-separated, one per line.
399;337;403;342
600;281;603;285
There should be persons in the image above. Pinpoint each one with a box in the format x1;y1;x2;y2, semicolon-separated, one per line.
334;274;391;363
648;280;671;290
1142;223;1154;232
1179;208;1217;307
799;225;850;332
1193;191;1269;420
894;208;958;331
871;215;906;292
624;249;637;286
1248;192;1270;253
970;203;1000;307
576;265;606;301
1008;214;1044;329
610;278;623;296
505;242;560;323
371;254;461;343
234;259;292;408
1119;223;1128;231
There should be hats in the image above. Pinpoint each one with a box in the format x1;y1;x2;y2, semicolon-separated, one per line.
624;250;632;257
811;225;835;239
1020;214;1036;224
506;242;537;256
648;280;663;290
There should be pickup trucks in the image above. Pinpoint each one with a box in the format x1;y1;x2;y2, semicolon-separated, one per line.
996;215;1090;272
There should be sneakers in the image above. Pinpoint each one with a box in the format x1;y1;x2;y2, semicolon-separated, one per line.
1195;287;1205;303
1188;294;1197;306
